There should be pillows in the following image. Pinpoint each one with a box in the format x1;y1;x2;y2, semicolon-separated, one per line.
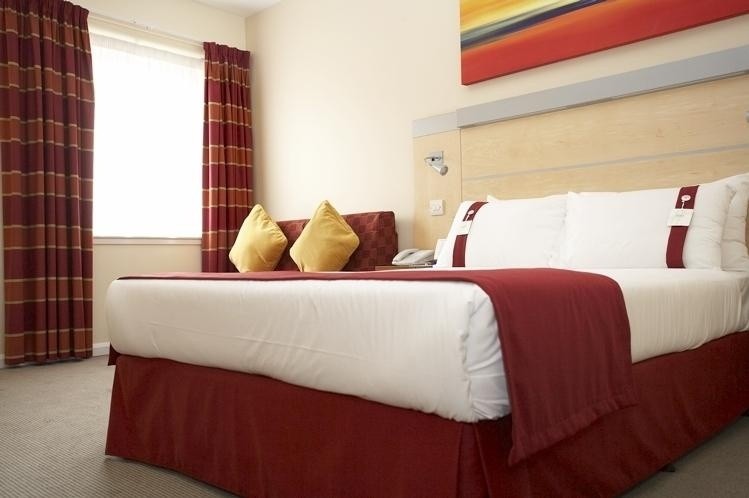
566;174;749;272
228;199;361;272
486;192;570;202
554;183;732;271
434;200;565;268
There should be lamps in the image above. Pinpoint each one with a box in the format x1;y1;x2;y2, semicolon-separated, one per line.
422;150;449;177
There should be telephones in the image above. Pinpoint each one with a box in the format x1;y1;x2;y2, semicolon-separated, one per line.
392;248;435;265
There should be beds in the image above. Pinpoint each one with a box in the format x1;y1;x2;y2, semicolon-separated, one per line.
101;266;749;497
